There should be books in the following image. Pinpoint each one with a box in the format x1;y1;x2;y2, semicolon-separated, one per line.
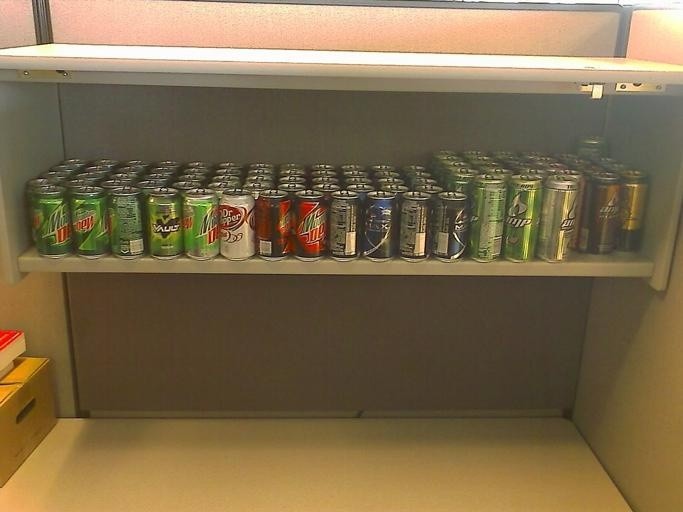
0;328;28;371
0;359;15;380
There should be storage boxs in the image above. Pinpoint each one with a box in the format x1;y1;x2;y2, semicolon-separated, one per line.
0;356;56;489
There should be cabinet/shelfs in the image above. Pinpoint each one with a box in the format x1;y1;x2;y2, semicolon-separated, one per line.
0;41;683;294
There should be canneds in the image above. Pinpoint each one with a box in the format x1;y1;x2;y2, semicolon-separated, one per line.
25;136;649;260
